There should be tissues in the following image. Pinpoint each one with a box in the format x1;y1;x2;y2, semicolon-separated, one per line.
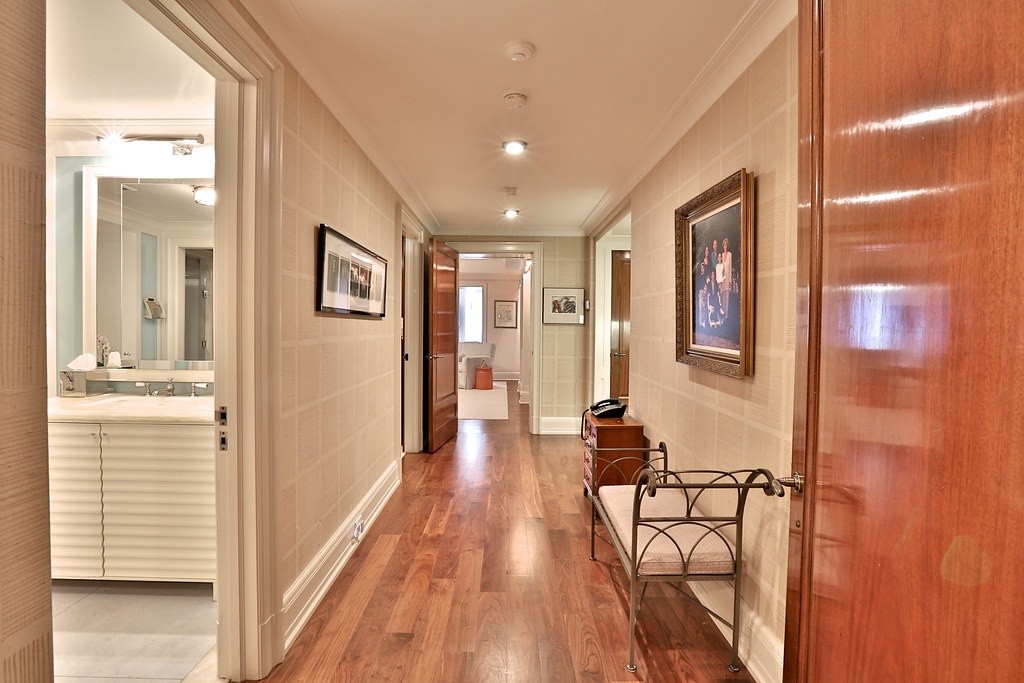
107;351;136;370
58;353;97;397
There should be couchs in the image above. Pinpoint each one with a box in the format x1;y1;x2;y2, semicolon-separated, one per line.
458;341;496;389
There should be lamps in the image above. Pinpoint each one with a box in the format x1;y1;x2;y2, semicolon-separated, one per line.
192;184;217;206
502;139;528;157
96;131;206;146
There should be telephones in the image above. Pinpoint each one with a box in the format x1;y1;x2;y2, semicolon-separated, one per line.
588;398;627;418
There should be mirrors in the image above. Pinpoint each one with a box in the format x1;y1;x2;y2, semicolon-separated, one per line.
81;162;217;371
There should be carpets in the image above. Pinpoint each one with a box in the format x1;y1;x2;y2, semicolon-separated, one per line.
458;380;509;420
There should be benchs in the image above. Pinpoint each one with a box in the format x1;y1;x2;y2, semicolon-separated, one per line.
589;442;776;674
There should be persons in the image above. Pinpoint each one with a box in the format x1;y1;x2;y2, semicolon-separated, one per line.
695;238;733;329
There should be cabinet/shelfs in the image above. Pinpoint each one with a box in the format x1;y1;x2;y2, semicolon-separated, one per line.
47;392;218;603
582;409;645;521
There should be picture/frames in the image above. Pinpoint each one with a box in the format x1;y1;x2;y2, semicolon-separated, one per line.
674;167;757;379
494;300;518;329
543;287;585;325
317;224;388;318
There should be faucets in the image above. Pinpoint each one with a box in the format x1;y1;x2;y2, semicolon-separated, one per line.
152;377;174;396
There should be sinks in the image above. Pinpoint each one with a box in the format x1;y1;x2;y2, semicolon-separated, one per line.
82;396;214;423
